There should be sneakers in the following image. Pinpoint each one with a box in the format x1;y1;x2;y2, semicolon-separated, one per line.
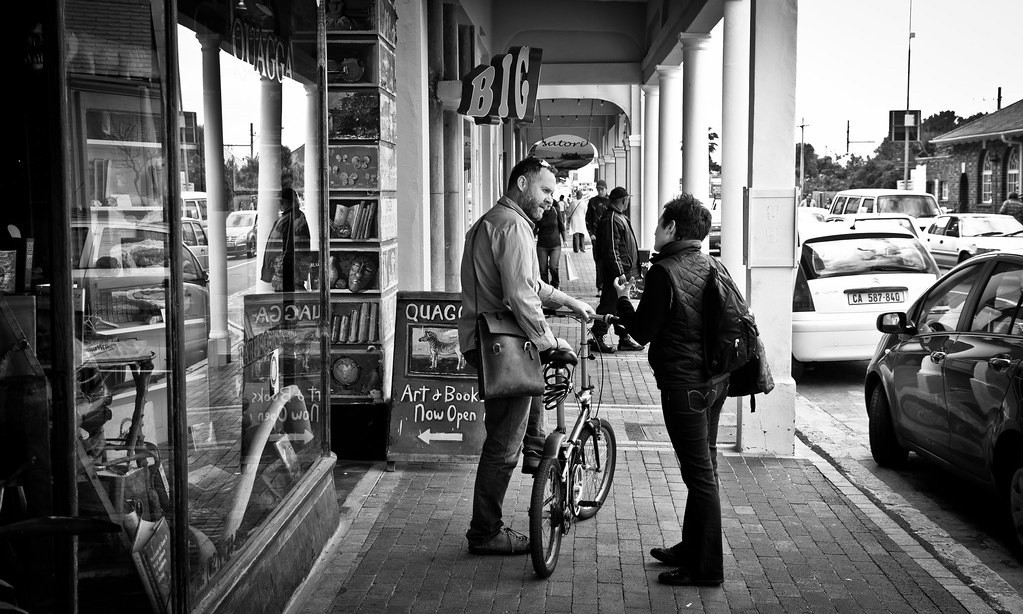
469;526;531;556
522;454;540;474
659;568;721;588
590;337;616;353
617;338;645;350
651;541;691;568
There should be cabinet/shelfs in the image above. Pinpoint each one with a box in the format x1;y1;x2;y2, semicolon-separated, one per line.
328;0;397;404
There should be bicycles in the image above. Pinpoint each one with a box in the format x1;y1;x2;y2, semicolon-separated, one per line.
524;309;628;577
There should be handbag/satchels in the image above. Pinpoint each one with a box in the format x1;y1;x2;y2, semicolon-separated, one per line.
726;337;772;399
565;247;579;282
566;220;570;230
476;312;546;400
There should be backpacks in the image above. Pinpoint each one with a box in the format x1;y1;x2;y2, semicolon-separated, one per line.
696;252;757;378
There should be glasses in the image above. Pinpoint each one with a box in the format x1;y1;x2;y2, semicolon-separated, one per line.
523;159;551;174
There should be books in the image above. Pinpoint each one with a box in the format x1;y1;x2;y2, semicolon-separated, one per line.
330;301;379;342
350;201;376;240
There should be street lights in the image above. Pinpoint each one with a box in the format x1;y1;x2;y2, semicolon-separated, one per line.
904;32;915;190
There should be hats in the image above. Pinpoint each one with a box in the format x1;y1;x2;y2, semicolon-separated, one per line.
609;187;632;200
596;181;607;189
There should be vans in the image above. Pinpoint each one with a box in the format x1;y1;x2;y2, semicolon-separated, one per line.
827;188;947;233
177;192;209;241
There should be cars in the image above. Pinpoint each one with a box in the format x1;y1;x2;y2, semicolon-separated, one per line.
65;206;260;426
708;179;1023;378
863;252;1023;567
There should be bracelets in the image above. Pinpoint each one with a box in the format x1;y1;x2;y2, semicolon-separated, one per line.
551;337;558;350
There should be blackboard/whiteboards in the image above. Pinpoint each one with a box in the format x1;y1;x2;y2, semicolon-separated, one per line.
242;293;323;462
386;291;486;461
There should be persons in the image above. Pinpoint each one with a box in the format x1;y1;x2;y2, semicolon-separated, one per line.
824;197;832;209
590;187;645;354
585;180;610;298
800;192;817;207
558;194;573;230
614;195;731;588
260;188;310;293
998;192;1023;216
568;191;588;253
458;157;596;555
533;199;567;290
348;255;378;292
236;195;258;210
